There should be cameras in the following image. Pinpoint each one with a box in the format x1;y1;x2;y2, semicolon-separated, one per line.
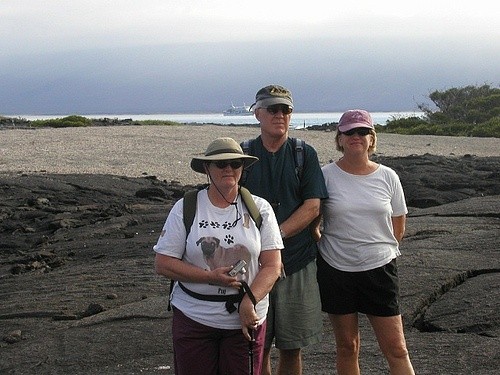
227;259;247;276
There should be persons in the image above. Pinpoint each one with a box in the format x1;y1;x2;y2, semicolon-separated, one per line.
238;84;328;375
153;138;285;375
313;109;415;375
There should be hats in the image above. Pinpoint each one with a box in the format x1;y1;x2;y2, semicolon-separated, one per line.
337;110;373;132
254;84;294;111
190;136;259;171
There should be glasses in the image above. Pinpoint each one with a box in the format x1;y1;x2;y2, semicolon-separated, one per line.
211;159;244;170
341;128;372;136
258;107;293;115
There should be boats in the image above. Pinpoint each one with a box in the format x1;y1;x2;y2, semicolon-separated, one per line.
223;102;254;116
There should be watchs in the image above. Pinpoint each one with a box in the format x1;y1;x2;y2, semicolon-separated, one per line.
280;229;286;238
